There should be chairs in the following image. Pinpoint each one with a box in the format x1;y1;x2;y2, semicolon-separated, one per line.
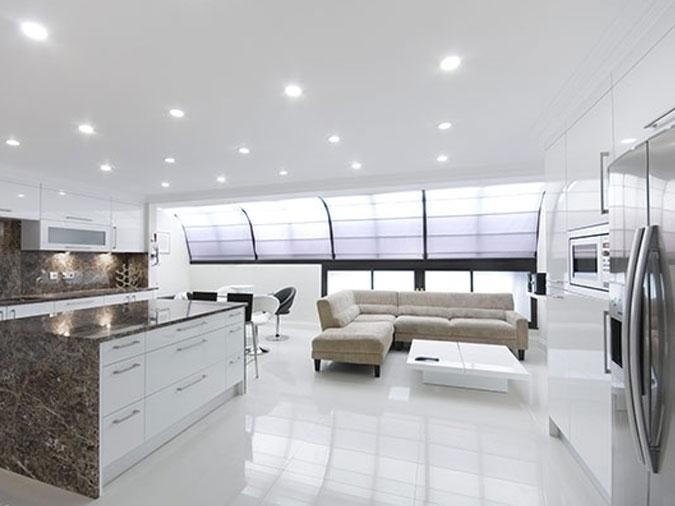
251;295;280;379
226;293;258;378
273;286;296;337
187;292;217;301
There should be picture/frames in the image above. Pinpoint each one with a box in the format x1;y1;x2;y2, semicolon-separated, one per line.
155;231;170;255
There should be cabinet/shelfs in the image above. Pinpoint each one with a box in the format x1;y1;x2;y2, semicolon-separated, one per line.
545;295;611;499
544;86;611;281
612;26;674;162
0;289;159;323
99;305;244;486
0;180;145;253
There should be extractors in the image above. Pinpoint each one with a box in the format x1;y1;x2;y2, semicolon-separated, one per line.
21;220;115;254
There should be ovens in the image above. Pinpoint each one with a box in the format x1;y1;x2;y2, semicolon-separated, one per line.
568;232;610;291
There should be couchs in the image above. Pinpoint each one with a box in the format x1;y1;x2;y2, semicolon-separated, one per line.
311;289;528;378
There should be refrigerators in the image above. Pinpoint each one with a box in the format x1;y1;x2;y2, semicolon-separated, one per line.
605;121;675;506
